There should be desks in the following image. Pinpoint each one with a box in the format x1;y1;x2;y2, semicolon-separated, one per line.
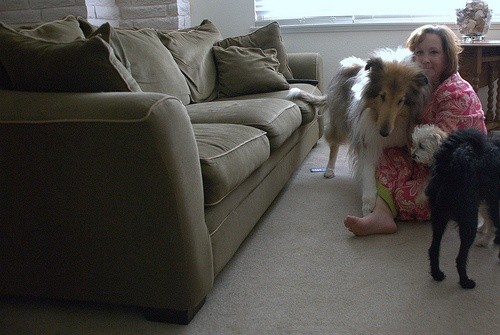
455;40;500;135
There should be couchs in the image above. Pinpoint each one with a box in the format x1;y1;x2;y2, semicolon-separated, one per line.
0;15;322;325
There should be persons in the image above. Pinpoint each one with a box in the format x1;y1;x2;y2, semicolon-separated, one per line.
344;25;487;236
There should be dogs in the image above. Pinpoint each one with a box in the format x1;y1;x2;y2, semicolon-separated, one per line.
422;126;500;290
283;43;434;217
408;120;494;246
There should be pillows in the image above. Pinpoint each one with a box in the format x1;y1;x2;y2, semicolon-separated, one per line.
1;21;141;93
75;22;132;73
211;44;290;101
215;20;294;81
